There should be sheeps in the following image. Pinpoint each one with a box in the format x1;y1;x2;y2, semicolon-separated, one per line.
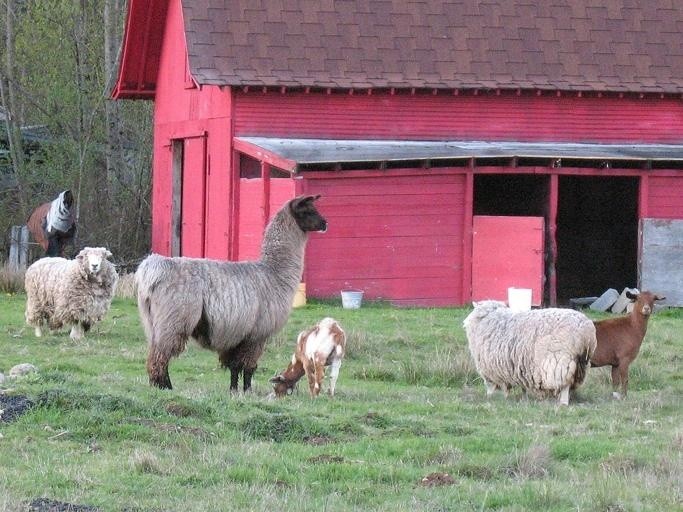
22;246;120;336
595;290;667;394
265;314;348;400
460;296;599;407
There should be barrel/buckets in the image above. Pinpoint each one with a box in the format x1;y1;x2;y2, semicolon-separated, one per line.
340;288;365;309
293;283;307;307
508;286;532;311
